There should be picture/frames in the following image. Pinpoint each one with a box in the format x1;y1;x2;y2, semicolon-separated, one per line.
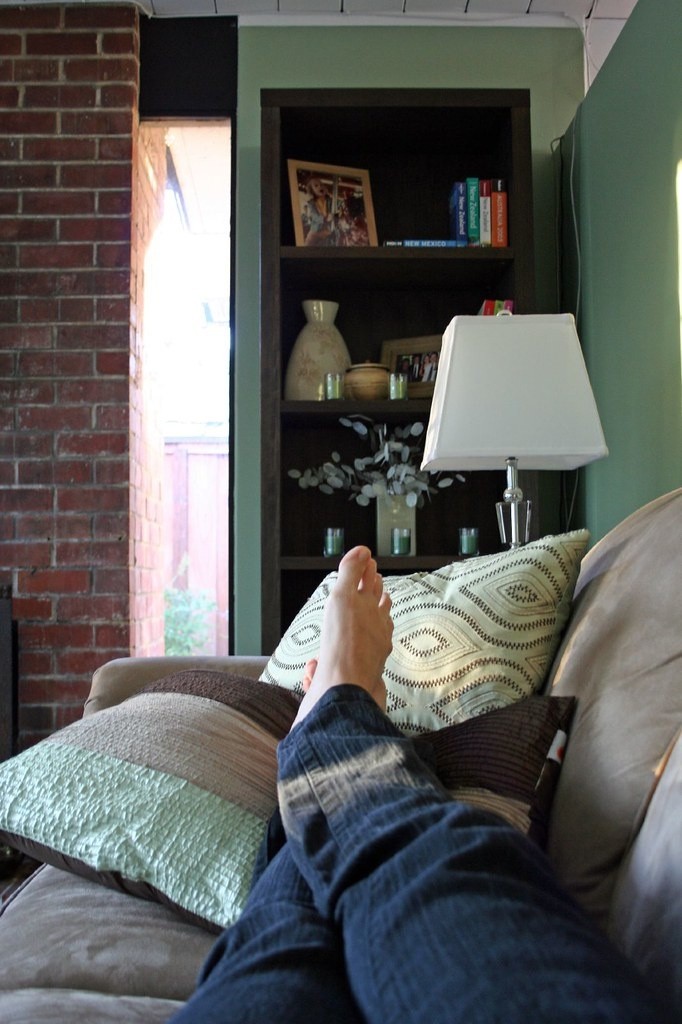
287;159;379;248
380;336;443;399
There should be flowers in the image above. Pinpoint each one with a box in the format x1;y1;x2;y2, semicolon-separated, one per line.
285;412;468;507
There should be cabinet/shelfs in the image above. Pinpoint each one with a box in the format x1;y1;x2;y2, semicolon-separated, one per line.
260;87;540;654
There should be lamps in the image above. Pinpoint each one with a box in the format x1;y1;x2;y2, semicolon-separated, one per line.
419;312;610;548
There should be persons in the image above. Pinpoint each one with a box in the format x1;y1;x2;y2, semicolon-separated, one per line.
170;546;666;1024
302;177;369;246
400;353;438;382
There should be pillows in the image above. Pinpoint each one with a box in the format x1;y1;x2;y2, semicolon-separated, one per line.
257;528;592;735
0;670;578;931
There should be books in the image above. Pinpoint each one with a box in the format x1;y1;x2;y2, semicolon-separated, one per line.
384;177;508;246
477;300;513;316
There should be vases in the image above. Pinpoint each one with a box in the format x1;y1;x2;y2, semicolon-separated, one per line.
375;495;416;558
284;298;353;402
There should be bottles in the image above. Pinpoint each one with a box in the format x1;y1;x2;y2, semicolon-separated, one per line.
284;299;352;401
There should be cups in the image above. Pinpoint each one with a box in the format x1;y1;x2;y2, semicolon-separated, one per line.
324;372;344;401
324;527;344;557
458;528;480;556
390;373;408;401
391;528;412;557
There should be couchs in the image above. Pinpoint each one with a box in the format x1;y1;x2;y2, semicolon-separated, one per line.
0;486;681;1023
